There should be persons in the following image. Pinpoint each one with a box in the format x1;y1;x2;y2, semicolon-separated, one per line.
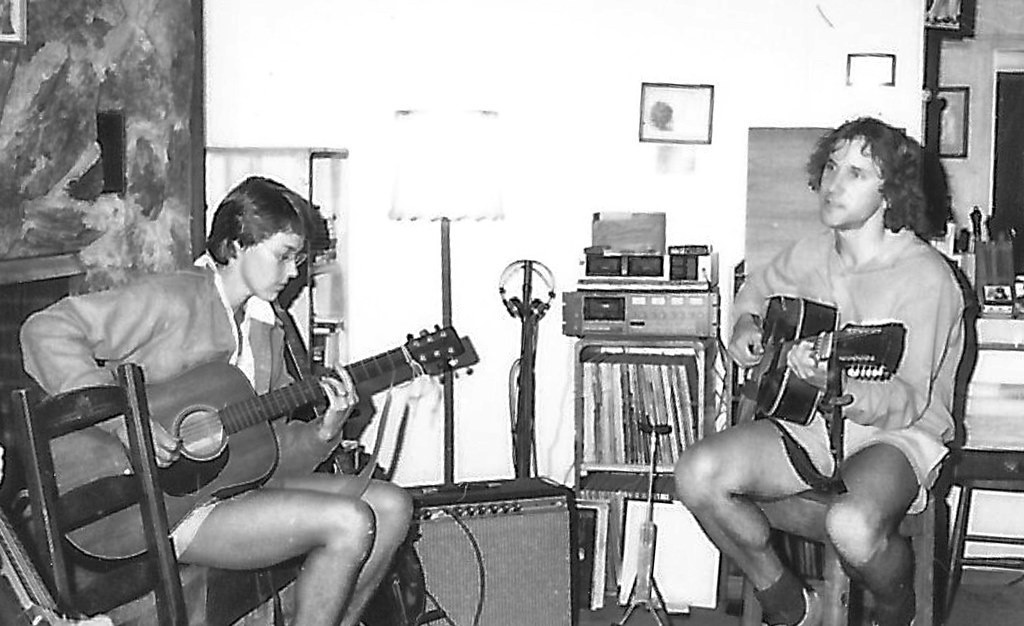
671;111;977;623
19;170;420;626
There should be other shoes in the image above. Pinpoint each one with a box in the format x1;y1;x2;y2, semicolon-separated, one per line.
761;586;823;626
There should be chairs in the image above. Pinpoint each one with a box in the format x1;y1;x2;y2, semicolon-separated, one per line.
10;359;195;625
740;249;985;626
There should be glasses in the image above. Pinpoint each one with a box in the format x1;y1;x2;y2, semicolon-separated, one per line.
258;239;308;266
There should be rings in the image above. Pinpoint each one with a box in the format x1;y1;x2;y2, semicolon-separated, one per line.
334;387;350;398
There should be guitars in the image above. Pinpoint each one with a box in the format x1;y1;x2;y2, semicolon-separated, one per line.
53;324;466;569
746;296;905;427
